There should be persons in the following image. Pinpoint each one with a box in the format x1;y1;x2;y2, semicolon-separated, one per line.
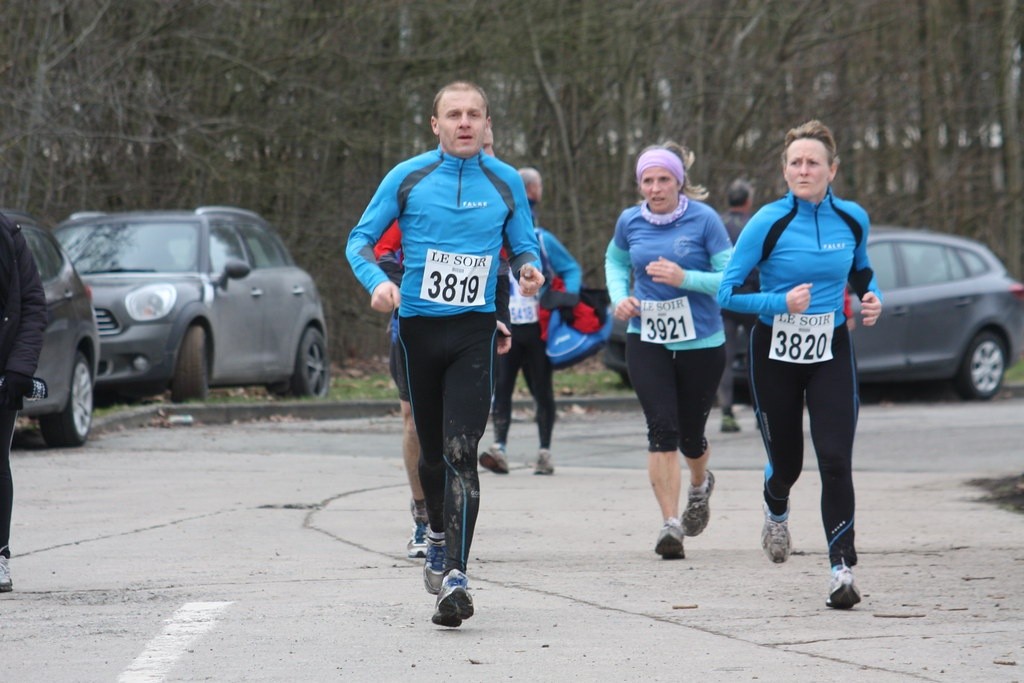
0;212;48;593
516;168;543;399
718;117;884;610
606;137;733;559
480;228;583;474
373;127;512;558
346;80;545;628
720;178;758;433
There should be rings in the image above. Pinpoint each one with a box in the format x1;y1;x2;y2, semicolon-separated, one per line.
527;289;530;294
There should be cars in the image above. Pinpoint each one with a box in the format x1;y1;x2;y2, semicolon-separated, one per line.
3;211;99;447
602;228;1024;402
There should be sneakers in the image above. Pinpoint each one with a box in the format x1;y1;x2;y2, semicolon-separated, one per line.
826;564;860;609
432;569;474;627
721;414;739;432
534;450;554;474
422;536;449;594
479;443;509;473
680;469;714;536
0;544;14;592
761;499;791;563
655;522;685;559
408;498;431;558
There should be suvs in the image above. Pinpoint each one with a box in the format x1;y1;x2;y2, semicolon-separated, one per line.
51;205;329;406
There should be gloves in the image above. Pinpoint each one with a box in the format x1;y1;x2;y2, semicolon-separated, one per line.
0;371;29;409
